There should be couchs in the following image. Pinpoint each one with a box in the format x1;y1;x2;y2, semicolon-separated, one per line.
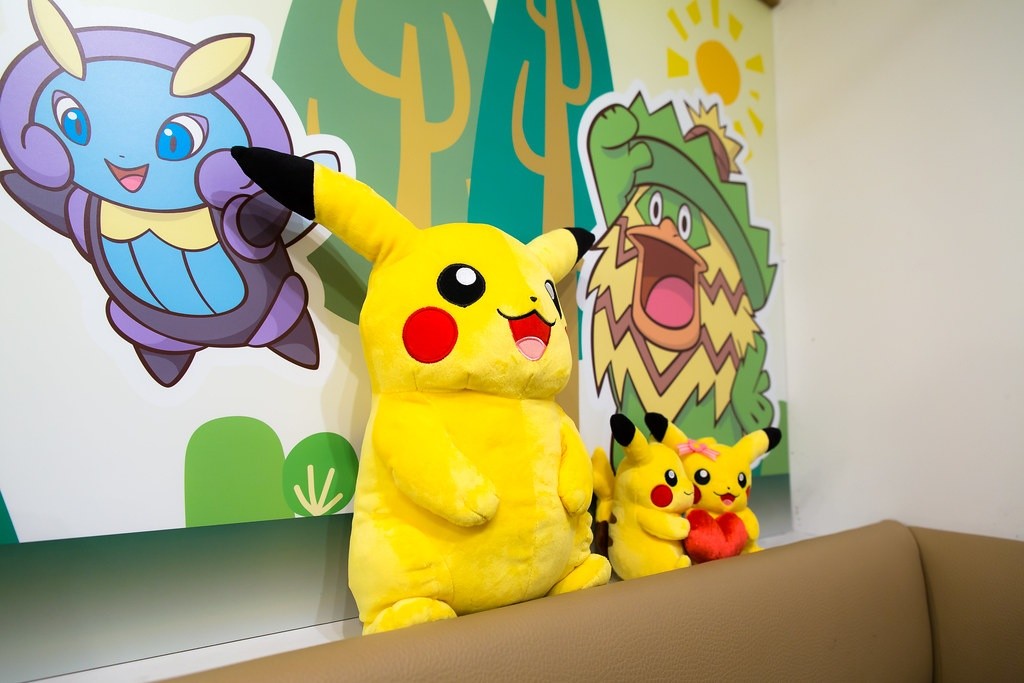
145;522;1024;683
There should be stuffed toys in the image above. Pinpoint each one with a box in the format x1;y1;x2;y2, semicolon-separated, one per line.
592;412;782;581
231;146;613;638
